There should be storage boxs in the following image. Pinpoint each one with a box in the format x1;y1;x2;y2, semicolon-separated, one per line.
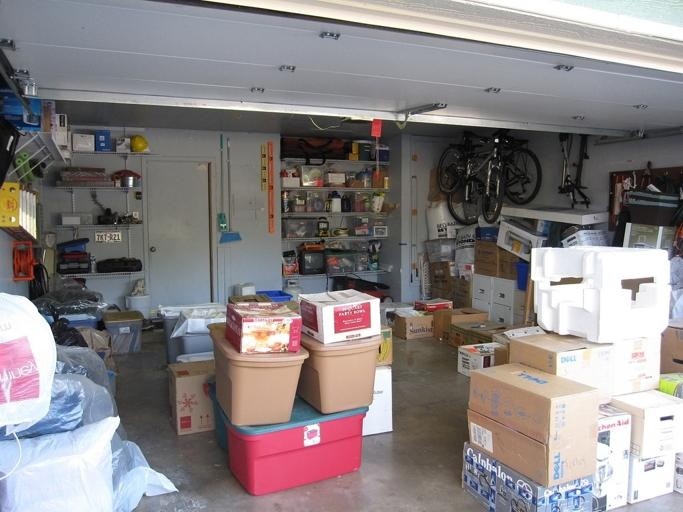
342;138;390;161
50;111;132;153
281;217;318;239
297;164;324;187
57;212;82;226
323;172;346;186
322;246;358;277
381;189;683;509
0;288;381;509
280;176;300;188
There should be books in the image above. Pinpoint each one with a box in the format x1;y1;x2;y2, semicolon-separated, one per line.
9;187;36;240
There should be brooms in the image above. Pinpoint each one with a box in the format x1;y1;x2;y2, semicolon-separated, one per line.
219;137;242;244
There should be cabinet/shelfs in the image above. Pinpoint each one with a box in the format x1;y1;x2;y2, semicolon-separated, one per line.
38;146;151;292
277;132;394;278
0;41;72;185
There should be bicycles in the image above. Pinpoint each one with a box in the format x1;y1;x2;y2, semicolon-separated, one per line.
446;129;541;227
437;128;511;224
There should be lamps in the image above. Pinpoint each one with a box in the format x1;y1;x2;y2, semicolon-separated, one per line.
398;103;447;116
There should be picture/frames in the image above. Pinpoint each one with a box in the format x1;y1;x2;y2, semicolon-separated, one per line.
370;225;388;238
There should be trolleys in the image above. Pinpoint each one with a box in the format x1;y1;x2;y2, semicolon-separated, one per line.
323;256;393;304
